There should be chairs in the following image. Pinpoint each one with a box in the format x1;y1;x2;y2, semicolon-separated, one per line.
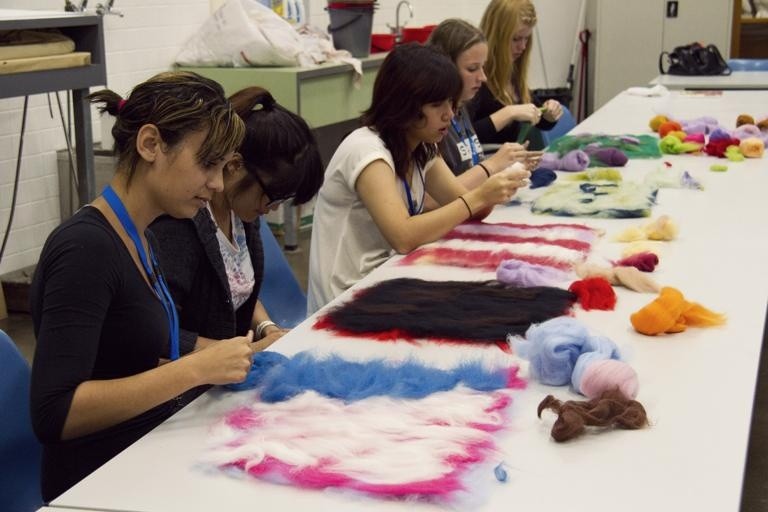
2;323;48;512
539;103;578;146
256;214;307;334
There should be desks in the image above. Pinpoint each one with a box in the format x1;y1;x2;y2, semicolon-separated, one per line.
38;90;768;512
0;10;109;208
649;68;767;90
172;52;391;252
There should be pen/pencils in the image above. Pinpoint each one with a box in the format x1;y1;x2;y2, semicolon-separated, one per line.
684;91;722;95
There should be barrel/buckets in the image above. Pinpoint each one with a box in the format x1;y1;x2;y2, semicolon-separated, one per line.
323;1;381;58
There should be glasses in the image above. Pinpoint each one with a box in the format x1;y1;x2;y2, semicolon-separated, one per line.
248;167;296;210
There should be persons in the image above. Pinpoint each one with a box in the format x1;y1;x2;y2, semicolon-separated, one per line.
423;18;543;213
145;87;324;357
464;0;564;155
28;69;253;506
306;42;531;318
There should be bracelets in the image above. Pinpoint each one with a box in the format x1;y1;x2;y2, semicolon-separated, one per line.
255;321;276;340
458;196;473;221
477;162;490;178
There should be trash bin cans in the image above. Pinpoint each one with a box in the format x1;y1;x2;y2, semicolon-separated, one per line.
531;87;576;147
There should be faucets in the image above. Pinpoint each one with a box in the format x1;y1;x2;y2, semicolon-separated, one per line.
395;1;413;47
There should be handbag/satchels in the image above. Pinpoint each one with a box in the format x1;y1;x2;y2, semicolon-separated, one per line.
667;43;729;76
